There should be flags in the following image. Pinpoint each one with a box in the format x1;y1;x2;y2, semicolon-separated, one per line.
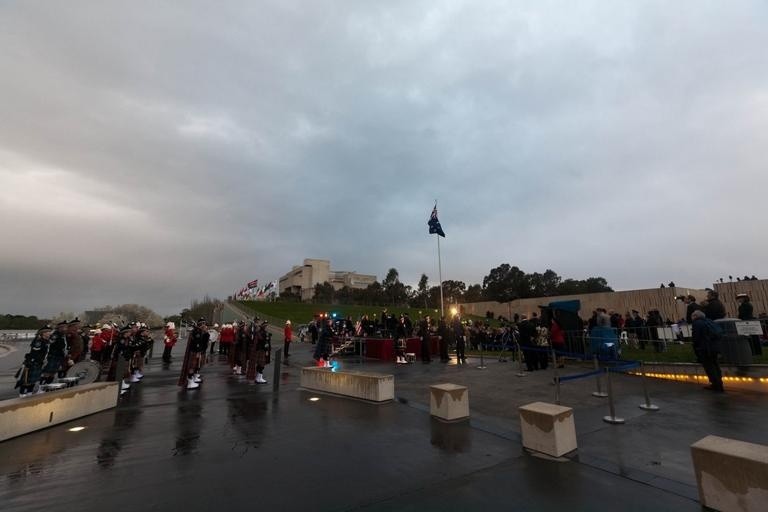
428;203;445;237
234;278;277;302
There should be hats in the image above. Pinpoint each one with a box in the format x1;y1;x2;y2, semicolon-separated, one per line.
69;316;81;326
285;319;292;325
37;325;53;334
93;315;269;338
55;319;70;329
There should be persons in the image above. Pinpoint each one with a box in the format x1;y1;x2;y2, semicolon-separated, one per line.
161;320;179;362
16;315;155;398
179;315;274;392
280;320;294;356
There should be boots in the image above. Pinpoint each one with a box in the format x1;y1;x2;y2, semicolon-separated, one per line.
118;363;269;397
400;355;408;364
323;360;334;368
395;355;403;364
